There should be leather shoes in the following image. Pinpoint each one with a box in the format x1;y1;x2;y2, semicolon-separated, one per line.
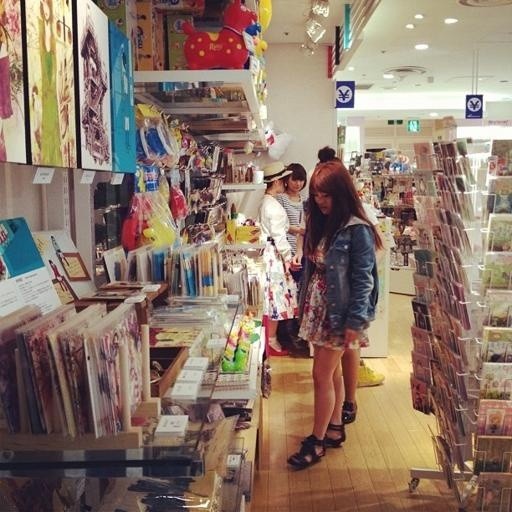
269;346;289;356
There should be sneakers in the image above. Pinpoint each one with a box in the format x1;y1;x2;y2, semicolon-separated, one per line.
357;360;384;386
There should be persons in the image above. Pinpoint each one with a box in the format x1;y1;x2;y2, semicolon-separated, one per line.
276;163;385;386
287;160;373;470
257;162;300;356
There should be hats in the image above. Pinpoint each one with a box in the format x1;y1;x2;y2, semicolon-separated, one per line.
263;162;293;182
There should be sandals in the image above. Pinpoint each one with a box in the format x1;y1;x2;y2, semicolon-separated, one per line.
287;401;357;466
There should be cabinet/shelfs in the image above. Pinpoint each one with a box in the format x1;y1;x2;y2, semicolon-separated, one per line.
0;283;266;511
133;69;270;190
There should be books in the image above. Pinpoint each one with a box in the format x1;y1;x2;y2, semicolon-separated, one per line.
410;141;512;512
0;300;159;435
